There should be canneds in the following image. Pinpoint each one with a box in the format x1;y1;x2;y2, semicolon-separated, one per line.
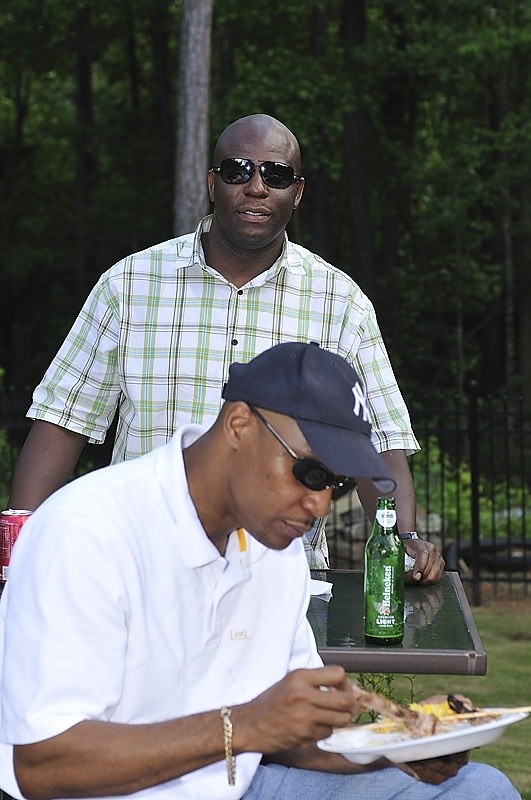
0;509;34;586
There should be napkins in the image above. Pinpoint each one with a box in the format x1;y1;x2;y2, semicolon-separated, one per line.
311;579;333;595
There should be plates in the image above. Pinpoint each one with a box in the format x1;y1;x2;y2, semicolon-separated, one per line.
318;705;530;766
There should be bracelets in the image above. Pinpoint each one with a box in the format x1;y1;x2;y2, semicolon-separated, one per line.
398;530;421;542
217;704;237;784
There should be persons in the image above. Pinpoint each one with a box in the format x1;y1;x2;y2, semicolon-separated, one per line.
6;115;445;585
1;342;522;800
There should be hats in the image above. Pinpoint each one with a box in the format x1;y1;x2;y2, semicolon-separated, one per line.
221;342;397;495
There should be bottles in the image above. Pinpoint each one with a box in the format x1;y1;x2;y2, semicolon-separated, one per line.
363;495;407;645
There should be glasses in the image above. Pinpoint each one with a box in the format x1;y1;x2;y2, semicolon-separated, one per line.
246;403;361;501
210;158;304;189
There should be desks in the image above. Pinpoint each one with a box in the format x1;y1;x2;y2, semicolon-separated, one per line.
307;568;488;676
